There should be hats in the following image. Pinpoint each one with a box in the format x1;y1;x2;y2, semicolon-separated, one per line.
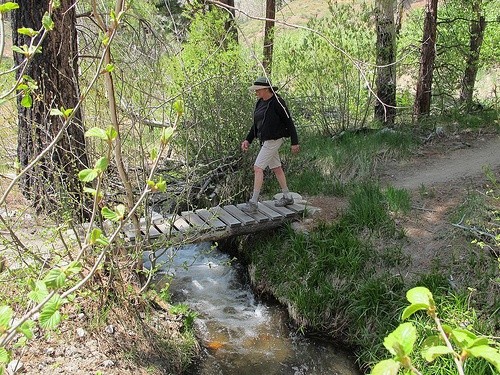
248;78;278;91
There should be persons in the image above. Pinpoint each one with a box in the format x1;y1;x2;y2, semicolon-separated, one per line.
236;75;302;213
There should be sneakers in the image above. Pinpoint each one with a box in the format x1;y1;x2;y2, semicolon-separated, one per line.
241;202;258;214
275;195;294;207
